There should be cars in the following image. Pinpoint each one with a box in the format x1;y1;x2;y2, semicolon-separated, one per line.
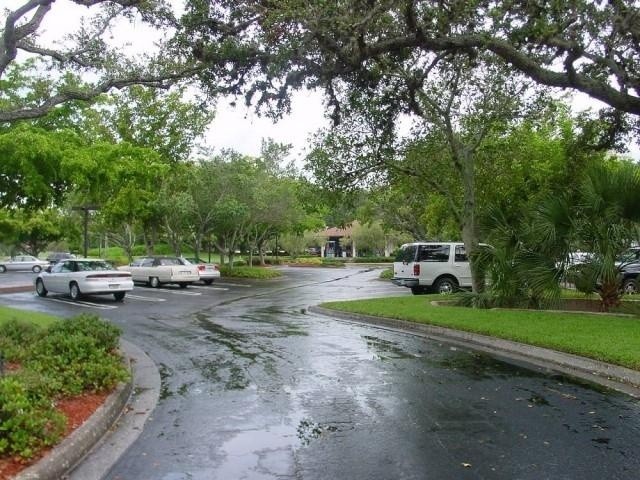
116;255;201;290
181;255;222;286
556;246;640;293
45;252;76;271
33;257;136;305
0;255;51;274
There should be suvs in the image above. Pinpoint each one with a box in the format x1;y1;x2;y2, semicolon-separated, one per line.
389;240;506;295
